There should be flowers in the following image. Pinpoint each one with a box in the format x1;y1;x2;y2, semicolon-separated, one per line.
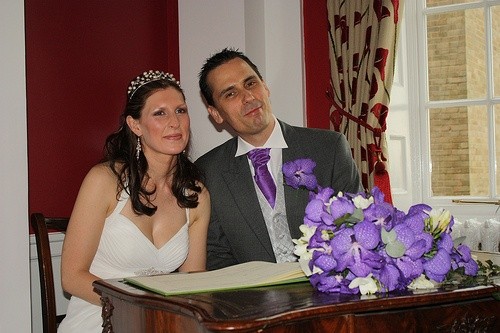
281;159;318;191
291;184;500;296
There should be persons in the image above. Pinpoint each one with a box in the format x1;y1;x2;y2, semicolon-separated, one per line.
55;67;213;333
192;47;363;273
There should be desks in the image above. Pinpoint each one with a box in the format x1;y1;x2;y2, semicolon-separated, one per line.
92;268;499;333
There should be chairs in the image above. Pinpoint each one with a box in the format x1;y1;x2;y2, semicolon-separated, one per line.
30;213;71;333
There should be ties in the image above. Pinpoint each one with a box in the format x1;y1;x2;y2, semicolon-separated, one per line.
247;148;276;209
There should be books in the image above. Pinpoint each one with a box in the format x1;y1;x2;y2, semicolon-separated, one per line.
123;259;310;297
